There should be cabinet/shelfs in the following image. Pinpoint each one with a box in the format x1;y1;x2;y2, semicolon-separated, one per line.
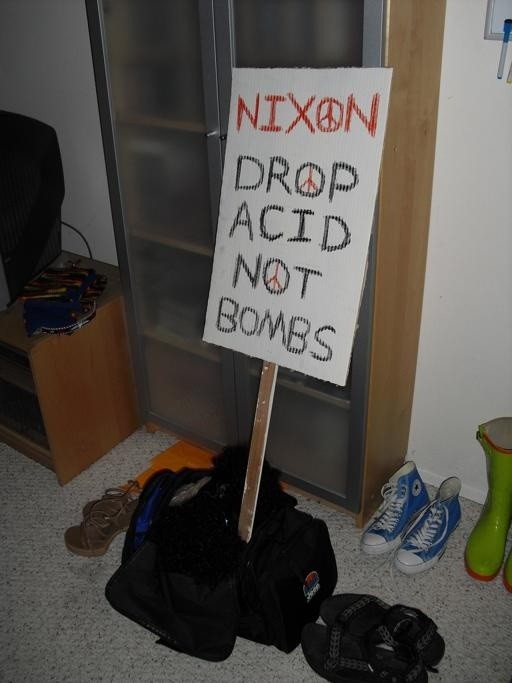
85;0;447;530
0;250;141;488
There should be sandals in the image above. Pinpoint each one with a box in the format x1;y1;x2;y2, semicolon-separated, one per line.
64;481;143;557
300;621;428;683
319;593;445;667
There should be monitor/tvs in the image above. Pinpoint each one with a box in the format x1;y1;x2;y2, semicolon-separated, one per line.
0;110;65;312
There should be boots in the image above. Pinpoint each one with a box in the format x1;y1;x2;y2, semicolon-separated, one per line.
394;477;461;574
465;417;512;592
361;461;430;554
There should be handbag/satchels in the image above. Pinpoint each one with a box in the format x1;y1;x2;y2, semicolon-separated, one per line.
105;443;337;662
